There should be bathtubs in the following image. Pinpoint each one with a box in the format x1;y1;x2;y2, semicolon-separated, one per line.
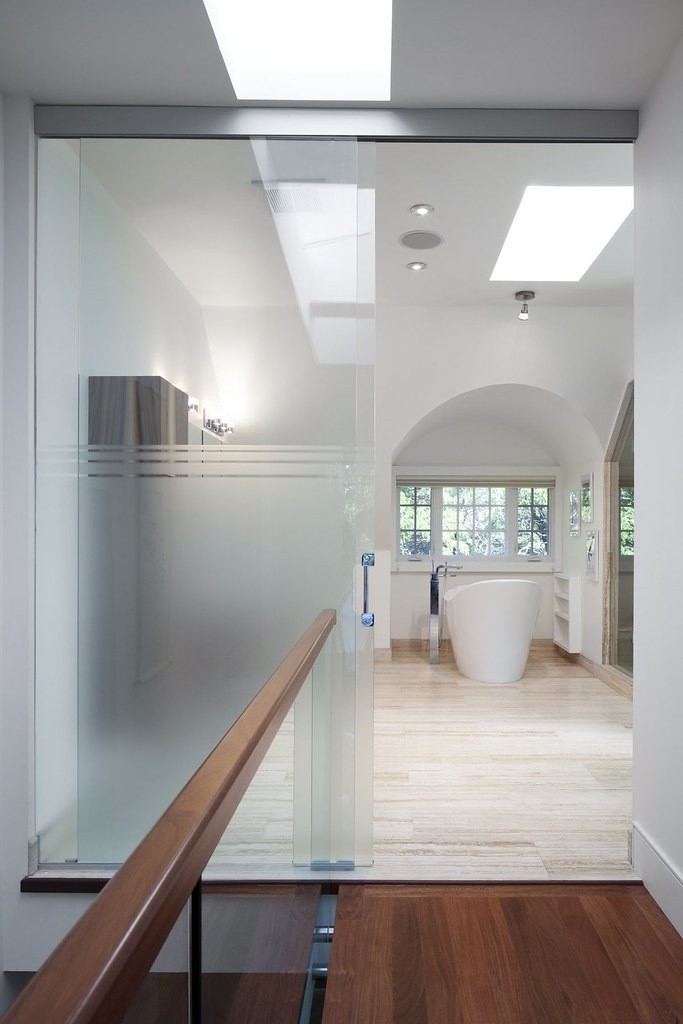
443;579;543;683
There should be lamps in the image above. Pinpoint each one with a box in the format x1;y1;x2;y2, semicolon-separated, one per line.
514;291;535;321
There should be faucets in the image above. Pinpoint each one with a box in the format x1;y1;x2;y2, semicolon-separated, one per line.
436;565;463;574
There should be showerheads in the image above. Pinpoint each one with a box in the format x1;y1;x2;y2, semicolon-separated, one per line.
444;562;447;574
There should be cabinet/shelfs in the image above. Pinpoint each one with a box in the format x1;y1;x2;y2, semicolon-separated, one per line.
553;572;582;654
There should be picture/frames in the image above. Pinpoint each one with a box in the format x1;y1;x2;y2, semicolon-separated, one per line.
584;530;599;581
567;472;594;537
